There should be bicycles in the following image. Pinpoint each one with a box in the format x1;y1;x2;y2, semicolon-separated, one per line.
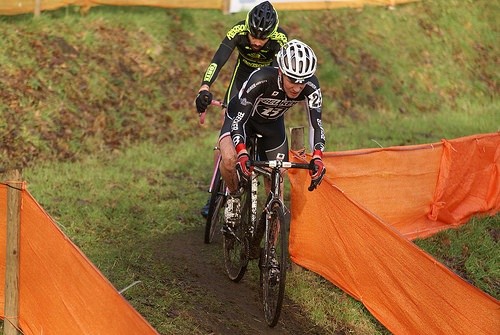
219;160;324;328
197;99;232;246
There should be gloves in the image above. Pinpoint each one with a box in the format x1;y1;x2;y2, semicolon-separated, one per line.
196;90;212;113
237;148;253;184
308;157;326;192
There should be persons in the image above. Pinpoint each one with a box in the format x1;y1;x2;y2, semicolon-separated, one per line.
219;39;324;283
195;0;287;218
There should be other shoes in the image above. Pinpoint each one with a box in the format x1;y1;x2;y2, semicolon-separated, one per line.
201;200;210;216
225;194;242;226
268;256;279;286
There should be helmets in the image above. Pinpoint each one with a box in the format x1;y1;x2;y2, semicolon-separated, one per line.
276;38;317;83
244;1;279;38
